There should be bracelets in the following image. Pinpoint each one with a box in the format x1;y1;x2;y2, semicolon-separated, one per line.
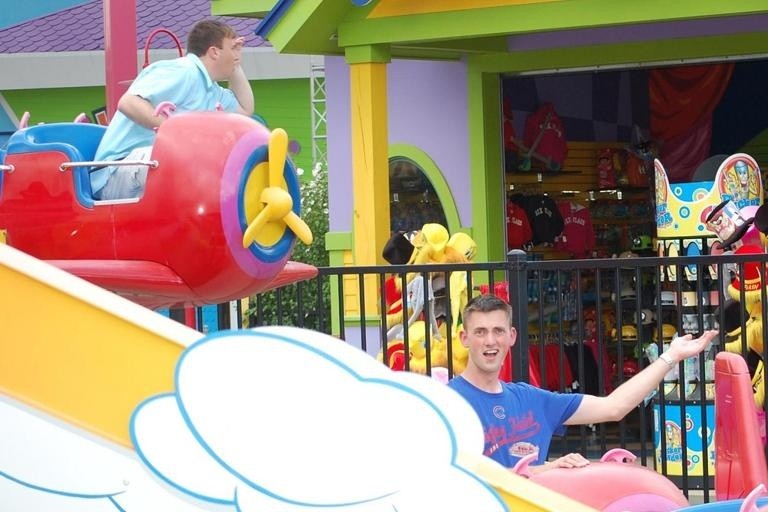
659;353;677;368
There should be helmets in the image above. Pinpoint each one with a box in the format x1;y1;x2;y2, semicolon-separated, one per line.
654;292;674;306
653;325;676;342
619;251;639;270
635;309;653;325
621;326;637;342
621;288;636;301
631;236;651;250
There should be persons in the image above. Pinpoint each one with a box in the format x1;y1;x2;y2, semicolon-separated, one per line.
447;293;720;476
91;23;255;201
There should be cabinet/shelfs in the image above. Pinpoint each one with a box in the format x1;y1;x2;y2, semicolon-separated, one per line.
508;170;653;345
